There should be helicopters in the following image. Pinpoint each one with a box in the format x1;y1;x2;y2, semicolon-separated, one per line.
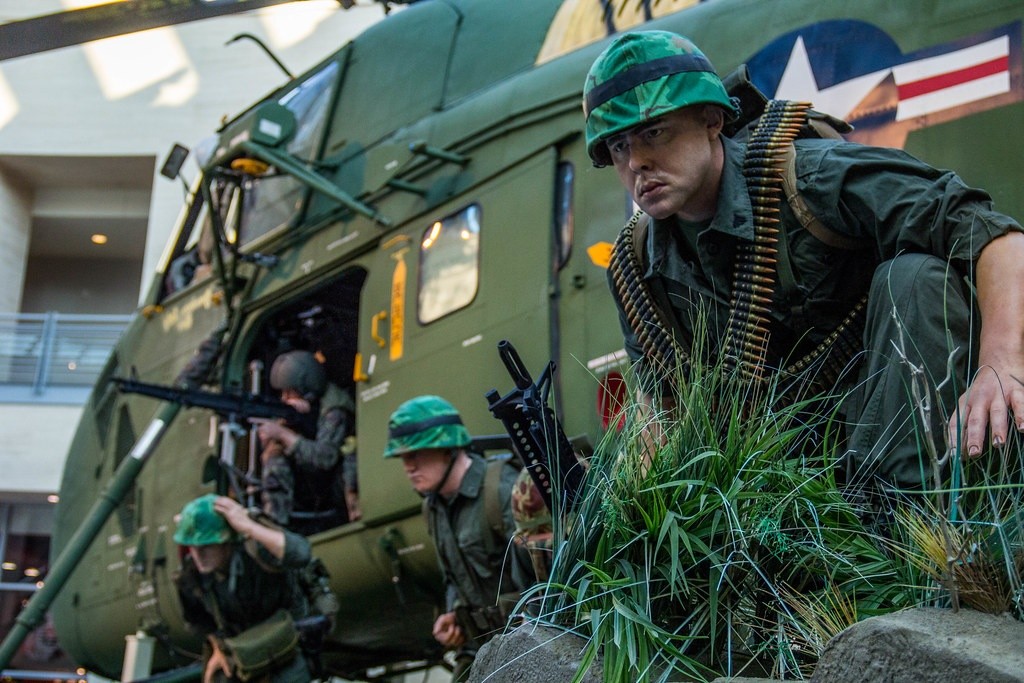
0;2;1024;683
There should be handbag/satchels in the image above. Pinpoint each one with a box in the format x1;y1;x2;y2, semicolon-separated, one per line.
222;613;302;678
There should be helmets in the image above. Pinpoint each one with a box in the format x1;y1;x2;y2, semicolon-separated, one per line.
382;396;470;460
173;493;233;547
581;30;742;168
271;352;324;404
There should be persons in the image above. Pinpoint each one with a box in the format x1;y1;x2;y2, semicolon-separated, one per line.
171;493;311;682
582;30;1024;657
383;394;541;682
247;350;355;528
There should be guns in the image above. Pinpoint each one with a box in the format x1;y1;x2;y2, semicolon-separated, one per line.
110;366;298;423
487;339;584;517
